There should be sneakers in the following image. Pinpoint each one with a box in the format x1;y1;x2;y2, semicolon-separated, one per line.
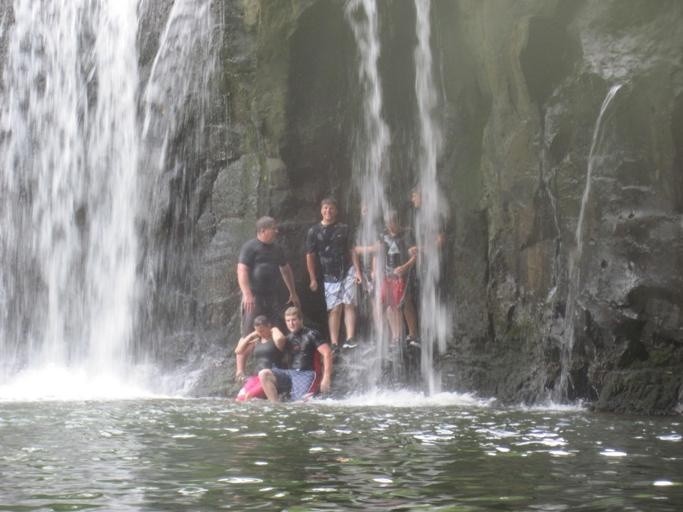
409;336;421;348
342;337;358;348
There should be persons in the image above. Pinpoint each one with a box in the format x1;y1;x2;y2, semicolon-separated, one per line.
235;315;287;401
354;186;446;350
259;306;332;403
306;198;363;355
238;216;302;338
